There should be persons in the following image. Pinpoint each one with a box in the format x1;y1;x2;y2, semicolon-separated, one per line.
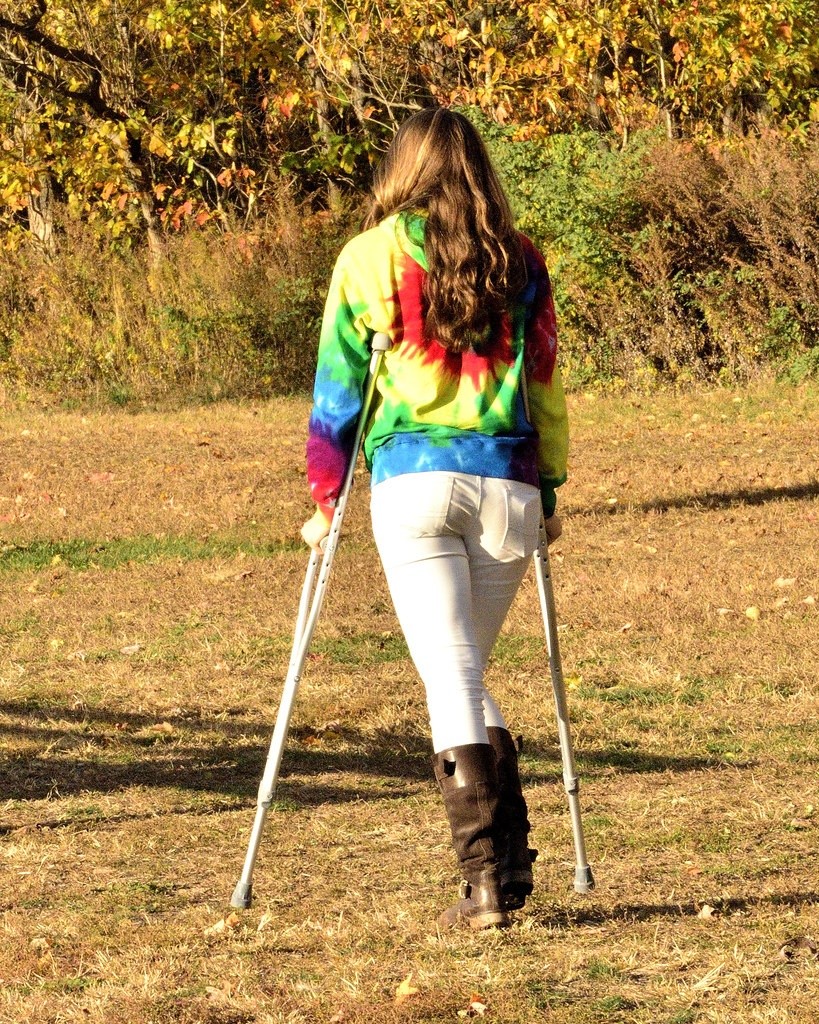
301;108;570;931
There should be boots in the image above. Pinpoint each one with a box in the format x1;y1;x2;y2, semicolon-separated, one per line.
486;726;538;911
430;743;508;931
